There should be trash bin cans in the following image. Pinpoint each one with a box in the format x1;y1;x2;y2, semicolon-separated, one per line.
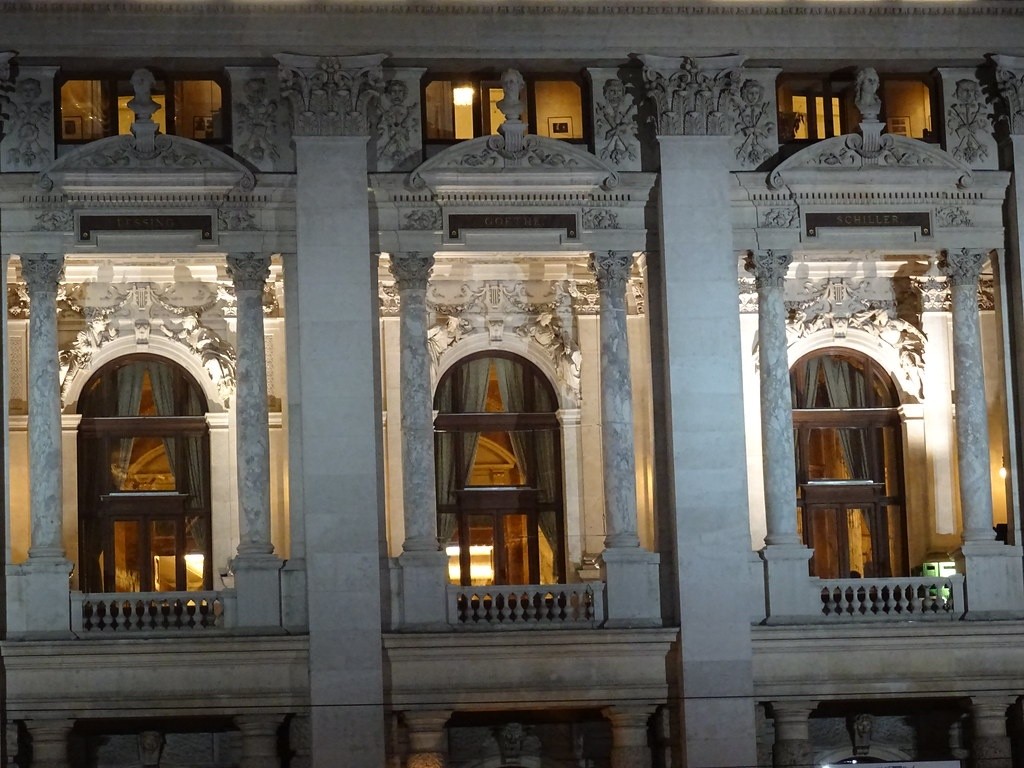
920;560;966;608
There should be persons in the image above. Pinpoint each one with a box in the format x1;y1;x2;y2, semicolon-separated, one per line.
496;70;525;113
854;713;873;735
753;311;825;373
855;67;879;103
160;314;235;402
59;315;117;410
502;724;522;742
141;732;159;752
849;308;928;399
128;69;161;113
516;312;582;399
427;314;471;360
